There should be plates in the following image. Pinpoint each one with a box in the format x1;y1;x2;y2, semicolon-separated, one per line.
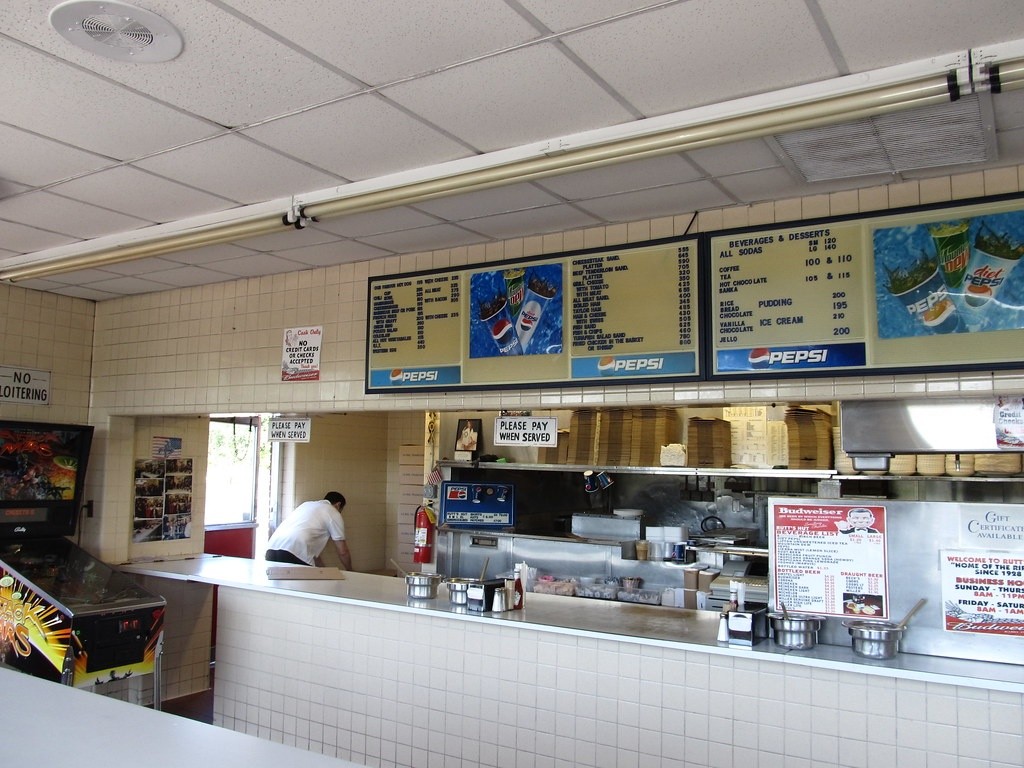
945;454;974;476
917;455;945;475
645;526;689;556
614;508;643;518
975;454;1021;477
889;454;916;476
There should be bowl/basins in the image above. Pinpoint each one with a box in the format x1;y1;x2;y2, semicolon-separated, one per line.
447;578;483;605
841;620;907;658
768;612;827;650
404;572;441;599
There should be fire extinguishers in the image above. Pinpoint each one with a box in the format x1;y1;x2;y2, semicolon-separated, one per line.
413;499;437;563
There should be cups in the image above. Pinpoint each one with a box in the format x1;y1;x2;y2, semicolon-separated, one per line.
957;245;1024;323
583;471;598;492
496;486;508;502
597;472;613;490
480;301;523;357
892;267;970;337
516;289;554;352
931;226;970;289
673;544;684;561
687;540;696;547
635;539;648;560
505;272;525;318
471;485;482;503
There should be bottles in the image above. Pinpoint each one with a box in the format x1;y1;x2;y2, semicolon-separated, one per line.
729;589;739;611
513;569;523;609
492;588;502;612
501;587;506;612
717;614;730;642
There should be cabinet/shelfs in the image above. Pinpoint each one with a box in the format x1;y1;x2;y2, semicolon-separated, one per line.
431;458;838;590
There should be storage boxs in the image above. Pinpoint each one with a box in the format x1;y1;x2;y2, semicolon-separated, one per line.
530;581;575;596
616;587;662;605
574;584;618;600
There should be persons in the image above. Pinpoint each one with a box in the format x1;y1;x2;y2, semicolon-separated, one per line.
459;420;476;451
264;491;353;570
